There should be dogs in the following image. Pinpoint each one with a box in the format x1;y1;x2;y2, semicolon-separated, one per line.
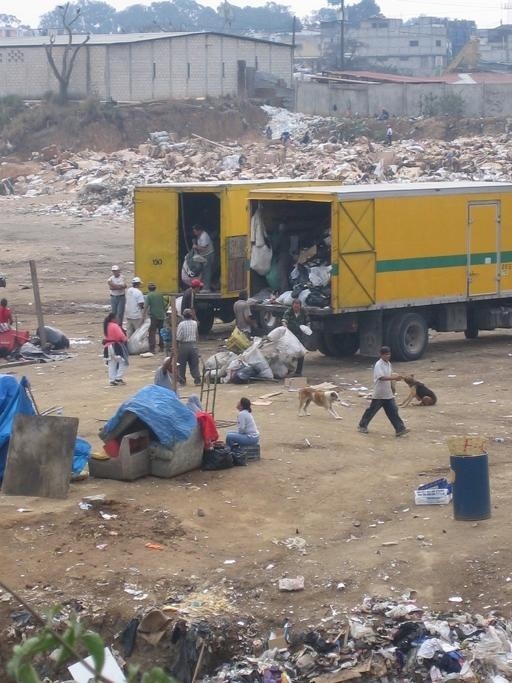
398;374;437;408
298;387;341;420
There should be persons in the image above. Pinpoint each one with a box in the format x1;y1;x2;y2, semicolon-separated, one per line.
266;126;273;139
105;264;127;325
376;107;389;121
225;397;259;451
282;298;310;379
272;219;291;291
357;344;412;437
153;350;175;391
232;289;257;340
191;224;215;294
125;276;144;340
0;298;15;334
180;278;201;316
174;308;203;387
386;125;394;145
101;314;128;385
144;284;167;355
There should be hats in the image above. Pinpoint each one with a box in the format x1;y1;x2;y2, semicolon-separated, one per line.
112;265;204;316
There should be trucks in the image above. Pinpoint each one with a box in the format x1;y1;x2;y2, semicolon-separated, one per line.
245;180;509;357
134;180;343;334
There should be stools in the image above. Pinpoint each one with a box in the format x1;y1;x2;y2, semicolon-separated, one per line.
240;440;260;462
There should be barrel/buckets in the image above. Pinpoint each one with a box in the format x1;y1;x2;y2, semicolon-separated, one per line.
449;451;491;520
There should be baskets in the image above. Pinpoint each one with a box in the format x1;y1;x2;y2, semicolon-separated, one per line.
415;477;453;505
